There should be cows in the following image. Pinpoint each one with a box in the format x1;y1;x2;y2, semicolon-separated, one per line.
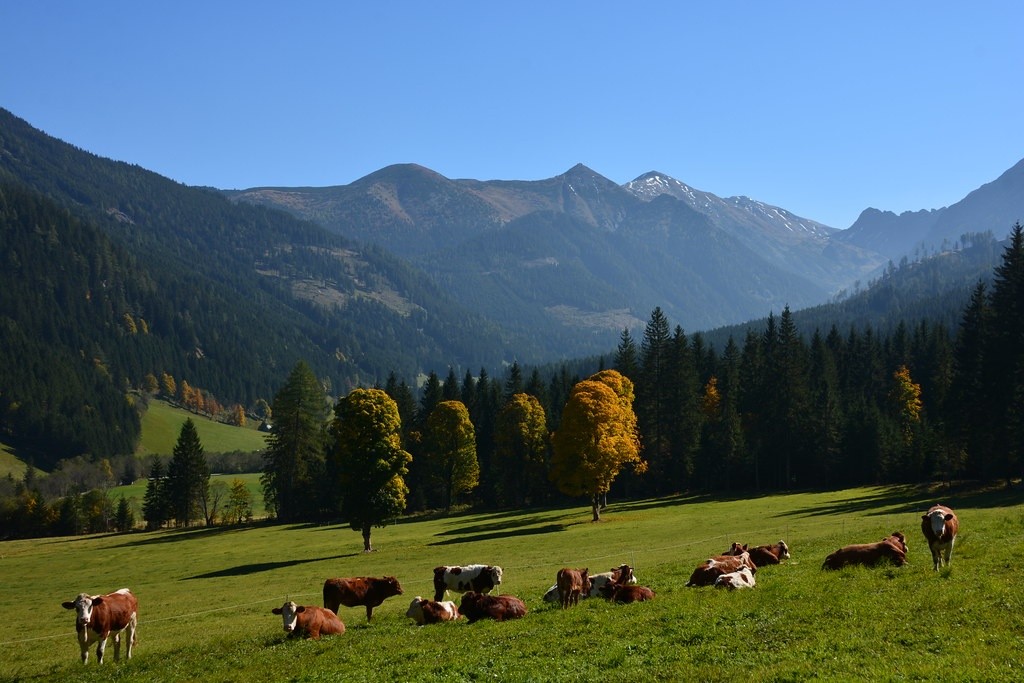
457;591;528;625
432;564;504;602
271;601;346;641
323;575;405;624
821;531;909;572
684;539;791;592
542;562;657;610
61;588;139;668
921;504;959;572
406;595;463;627
